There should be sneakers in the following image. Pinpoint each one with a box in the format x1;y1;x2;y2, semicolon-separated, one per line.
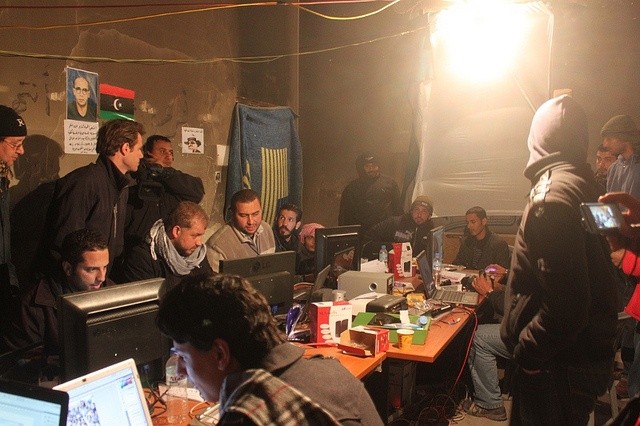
462;398;507;421
616;379;638;397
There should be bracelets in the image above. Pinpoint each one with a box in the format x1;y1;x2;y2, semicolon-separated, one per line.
483;290;494;297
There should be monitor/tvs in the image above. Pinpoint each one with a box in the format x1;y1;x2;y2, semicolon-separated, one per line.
426;226;444;277
219;250;296;317
314;224;362;290
59;277;172;386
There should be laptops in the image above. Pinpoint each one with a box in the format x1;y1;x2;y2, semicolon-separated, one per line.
415;249;479;306
0;380;69;426
51;358;154;426
295;265;331;318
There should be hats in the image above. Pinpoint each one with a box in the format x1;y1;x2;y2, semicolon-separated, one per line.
0;104;27;137
357;152;381;166
299;223;325;244
600;114;638;136
410;196;433;214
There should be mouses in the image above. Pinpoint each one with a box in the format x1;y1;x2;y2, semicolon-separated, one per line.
485;268;496;273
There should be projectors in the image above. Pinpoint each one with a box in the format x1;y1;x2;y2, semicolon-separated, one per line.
337;270;394;299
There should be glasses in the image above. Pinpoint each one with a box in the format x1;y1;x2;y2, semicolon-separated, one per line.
5;140;24;151
74;87;89;94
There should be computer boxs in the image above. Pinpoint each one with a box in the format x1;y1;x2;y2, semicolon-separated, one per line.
388;360;416;408
364;376;387;426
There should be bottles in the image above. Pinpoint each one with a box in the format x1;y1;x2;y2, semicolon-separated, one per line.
164;346;192;426
379;244;390;271
431;253;442;286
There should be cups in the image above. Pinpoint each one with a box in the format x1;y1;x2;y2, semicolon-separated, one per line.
396;327;416;351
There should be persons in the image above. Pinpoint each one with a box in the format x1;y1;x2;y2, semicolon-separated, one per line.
154;265;387;426
499;92;612;425
216;173;220;180
361;195;434;258
610;247;640;399
599;114;640;211
0;104;27;318
450;206;512;272
204;189;277;273
461;263;513;422
37;117;145;275
598;192;639;241
0;227;116;363
183;137;202;155
338;152;404;229
118;135;205;261
112;200;213;284
298;222;326;262
67;76;98;122
271;203;315;282
594;143;621;189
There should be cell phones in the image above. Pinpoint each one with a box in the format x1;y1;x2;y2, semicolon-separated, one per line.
580;201;626;234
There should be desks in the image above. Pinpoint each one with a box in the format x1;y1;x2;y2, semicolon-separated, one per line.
143;341;388;426
290;262;506;422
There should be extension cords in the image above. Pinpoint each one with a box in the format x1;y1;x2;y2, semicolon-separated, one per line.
387;409;404;424
